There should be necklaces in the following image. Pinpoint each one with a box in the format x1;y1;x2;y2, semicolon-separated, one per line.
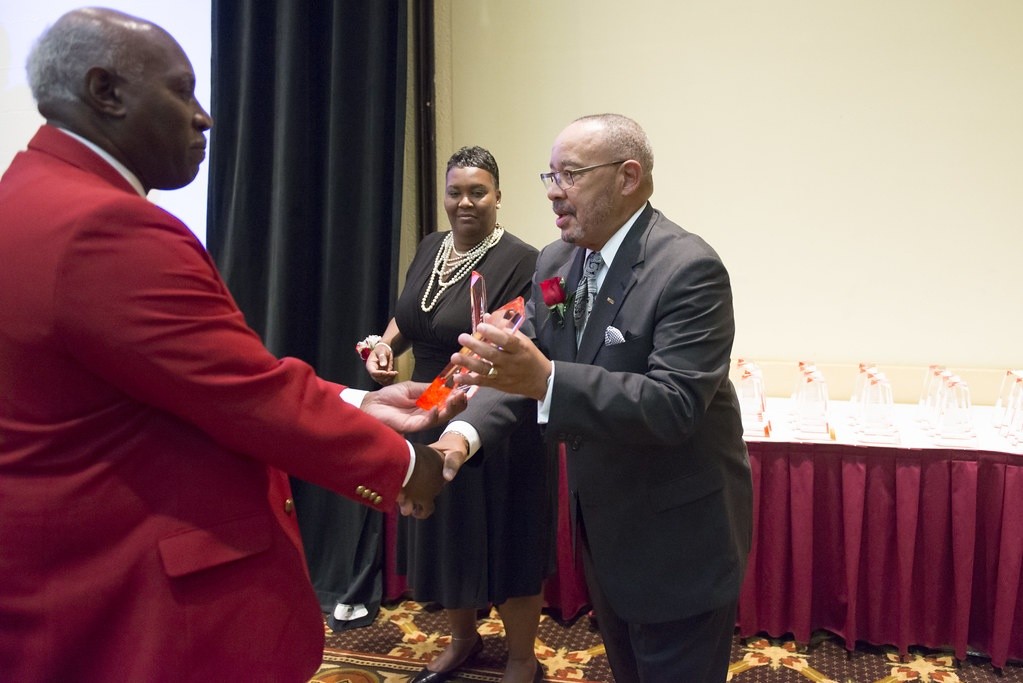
421;222;504;313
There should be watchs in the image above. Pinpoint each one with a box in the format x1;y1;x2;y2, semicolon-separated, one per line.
539;373;551;402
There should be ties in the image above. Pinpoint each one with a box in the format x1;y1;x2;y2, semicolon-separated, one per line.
573;251;605;343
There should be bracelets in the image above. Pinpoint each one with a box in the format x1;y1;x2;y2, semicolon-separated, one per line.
452;431;468;451
357;334;393;360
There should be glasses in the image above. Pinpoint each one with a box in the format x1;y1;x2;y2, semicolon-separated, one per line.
539;159;627;191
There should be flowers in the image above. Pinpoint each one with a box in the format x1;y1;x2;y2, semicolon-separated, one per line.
538;278;573;332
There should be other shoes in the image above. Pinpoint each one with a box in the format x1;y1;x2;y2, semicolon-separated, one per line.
411;632;485;683
499;658;545;683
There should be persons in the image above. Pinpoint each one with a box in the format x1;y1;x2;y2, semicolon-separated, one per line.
399;115;755;683
1;3;468;683
365;145;560;683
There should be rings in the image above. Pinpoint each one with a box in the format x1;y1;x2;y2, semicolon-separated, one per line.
379;354;384;357
485;364;499;380
